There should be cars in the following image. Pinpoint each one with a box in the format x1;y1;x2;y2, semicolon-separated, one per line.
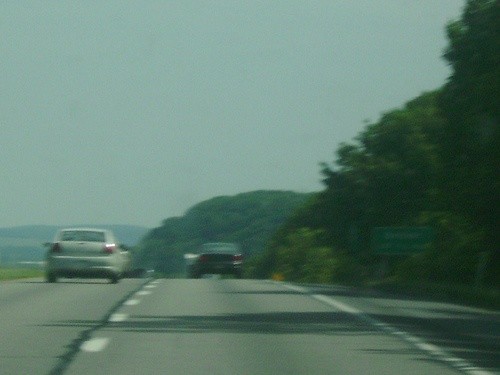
192;242;244;278
42;228;128;283
